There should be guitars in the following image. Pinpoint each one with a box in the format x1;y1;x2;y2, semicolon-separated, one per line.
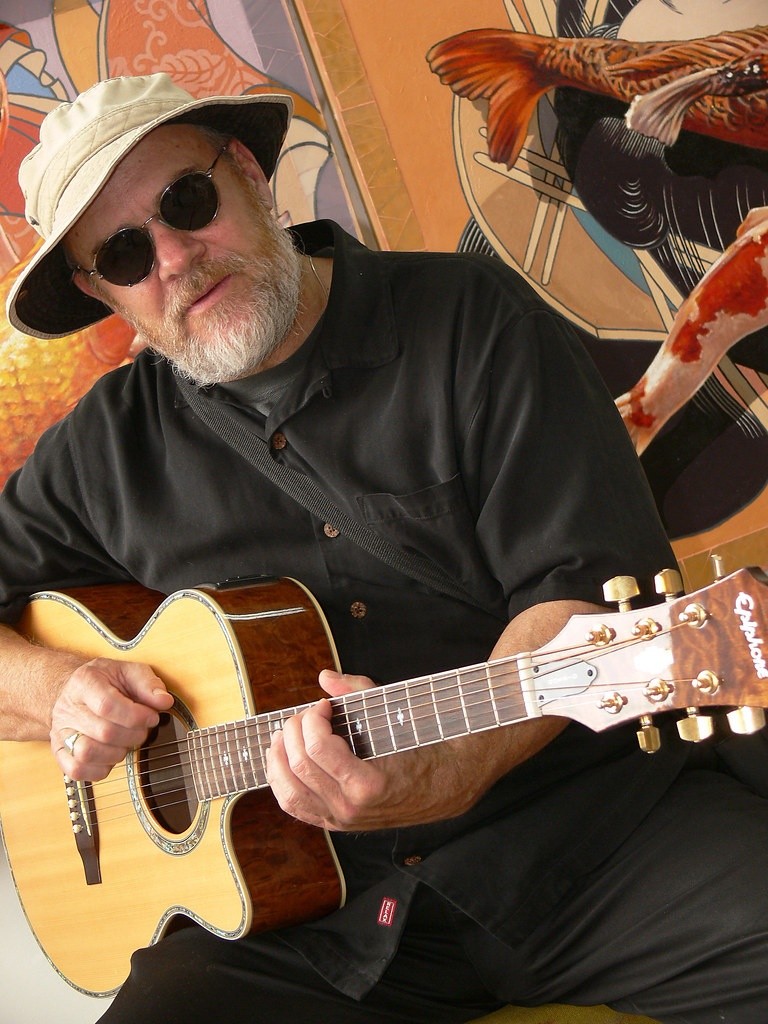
0;553;768;999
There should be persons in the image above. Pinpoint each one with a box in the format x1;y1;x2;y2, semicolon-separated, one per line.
0;72;768;1024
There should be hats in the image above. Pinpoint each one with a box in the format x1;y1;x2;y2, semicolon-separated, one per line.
5;72;294;343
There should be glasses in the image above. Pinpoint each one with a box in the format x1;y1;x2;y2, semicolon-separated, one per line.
75;141;224;285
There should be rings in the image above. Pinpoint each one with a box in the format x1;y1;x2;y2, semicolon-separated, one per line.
63;732;85;754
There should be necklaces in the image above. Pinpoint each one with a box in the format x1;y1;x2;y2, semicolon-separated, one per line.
306;252;329;303
